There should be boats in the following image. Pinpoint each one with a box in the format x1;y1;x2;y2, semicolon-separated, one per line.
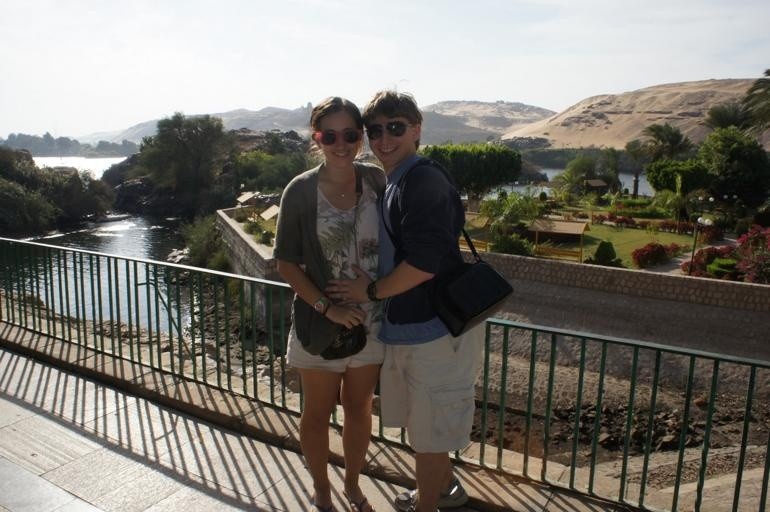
84;213;133;223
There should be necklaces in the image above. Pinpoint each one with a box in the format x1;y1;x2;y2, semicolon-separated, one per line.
325;168;360;200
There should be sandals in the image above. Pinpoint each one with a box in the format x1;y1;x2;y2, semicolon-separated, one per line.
310;485;377;512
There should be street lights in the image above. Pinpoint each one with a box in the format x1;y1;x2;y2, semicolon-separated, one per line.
698;196;716;215
688;218;714;276
723;194;738;234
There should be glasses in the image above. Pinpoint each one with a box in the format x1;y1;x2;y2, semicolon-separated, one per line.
318;129;360;146
365;120;408;141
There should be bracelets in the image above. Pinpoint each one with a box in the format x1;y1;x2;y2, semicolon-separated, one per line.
321;300;334;316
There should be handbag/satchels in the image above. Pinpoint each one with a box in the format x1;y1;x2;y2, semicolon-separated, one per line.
426;257;516;340
314;315;369;362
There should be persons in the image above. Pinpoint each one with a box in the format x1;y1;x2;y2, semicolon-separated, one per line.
275;97;390;509
324;90;487;511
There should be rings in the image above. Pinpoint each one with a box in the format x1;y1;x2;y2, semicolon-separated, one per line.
342;293;347;300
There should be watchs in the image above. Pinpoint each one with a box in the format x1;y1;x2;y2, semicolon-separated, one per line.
366;279;385;303
313;293;329;312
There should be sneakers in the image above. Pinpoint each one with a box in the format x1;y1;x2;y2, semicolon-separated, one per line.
392;475;470;512
